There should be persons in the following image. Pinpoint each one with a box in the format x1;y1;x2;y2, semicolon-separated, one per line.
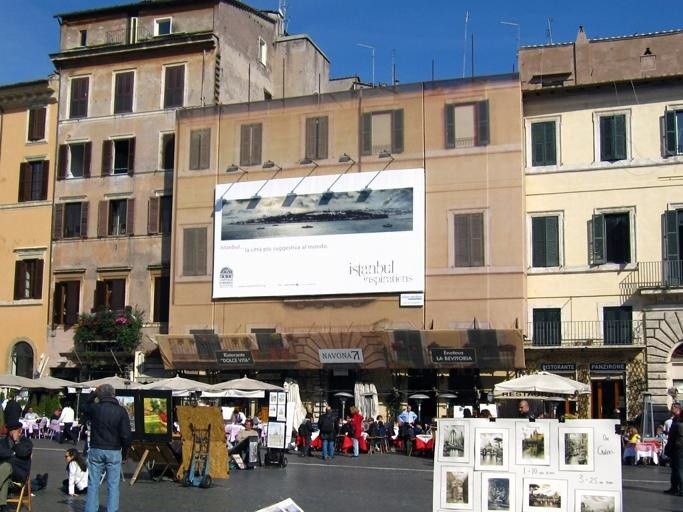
0;392;91;459
169;407;268;471
571;444;587;464
663;402;682;496
292;402;435;461
78;382;131;512
0;420;33;512
440;399;579;418
614;407;676;467
61;447;88;498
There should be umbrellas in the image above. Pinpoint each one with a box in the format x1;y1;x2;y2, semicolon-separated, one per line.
493;370;579;402
353;380;378;422
539;371;592;398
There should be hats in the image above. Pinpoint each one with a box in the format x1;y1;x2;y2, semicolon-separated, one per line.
5;421;22;428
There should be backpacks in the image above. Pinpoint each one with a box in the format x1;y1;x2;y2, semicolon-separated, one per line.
298;421;309;437
321;415;332;434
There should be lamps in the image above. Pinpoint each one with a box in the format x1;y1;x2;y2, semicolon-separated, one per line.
300;158;320;167
379;149;396;161
226;163;249;175
339;153;356;164
264;160;284;170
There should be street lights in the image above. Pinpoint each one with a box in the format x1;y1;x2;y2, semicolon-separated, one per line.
355;43;375;88
499;22;520;50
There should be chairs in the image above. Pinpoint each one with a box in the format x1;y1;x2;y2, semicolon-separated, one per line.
295;428;360;454
7;454;35;512
19;415;60;442
625;442;659;467
415;434;433;456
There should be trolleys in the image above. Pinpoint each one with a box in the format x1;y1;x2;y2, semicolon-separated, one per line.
183;422;212;488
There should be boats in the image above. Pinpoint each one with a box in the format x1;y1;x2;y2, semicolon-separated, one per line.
302;224;314;228
383;222;392;227
257;224;279;229
